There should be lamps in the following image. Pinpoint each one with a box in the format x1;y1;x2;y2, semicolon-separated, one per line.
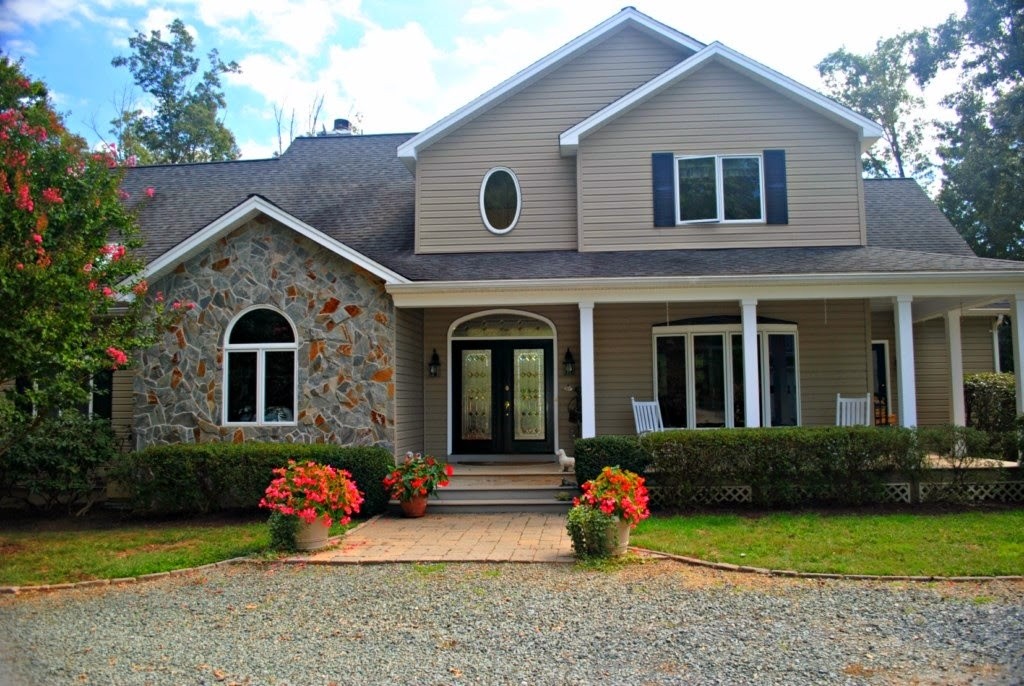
563;348;575;376
429;348;441;378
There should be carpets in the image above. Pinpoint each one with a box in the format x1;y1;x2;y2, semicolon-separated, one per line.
457;461;556;466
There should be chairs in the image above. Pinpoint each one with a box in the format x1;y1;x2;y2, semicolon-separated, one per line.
836;393;870;428
631;397;664;437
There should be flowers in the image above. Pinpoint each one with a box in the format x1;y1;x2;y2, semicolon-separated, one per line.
258;459;365;553
382;451;453;503
565;465;650;560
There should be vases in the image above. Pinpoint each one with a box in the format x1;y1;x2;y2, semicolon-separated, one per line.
583;515;631;556
401;493;427;516
293;515;329;549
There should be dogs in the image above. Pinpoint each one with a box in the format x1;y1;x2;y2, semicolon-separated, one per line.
556;448;576;472
